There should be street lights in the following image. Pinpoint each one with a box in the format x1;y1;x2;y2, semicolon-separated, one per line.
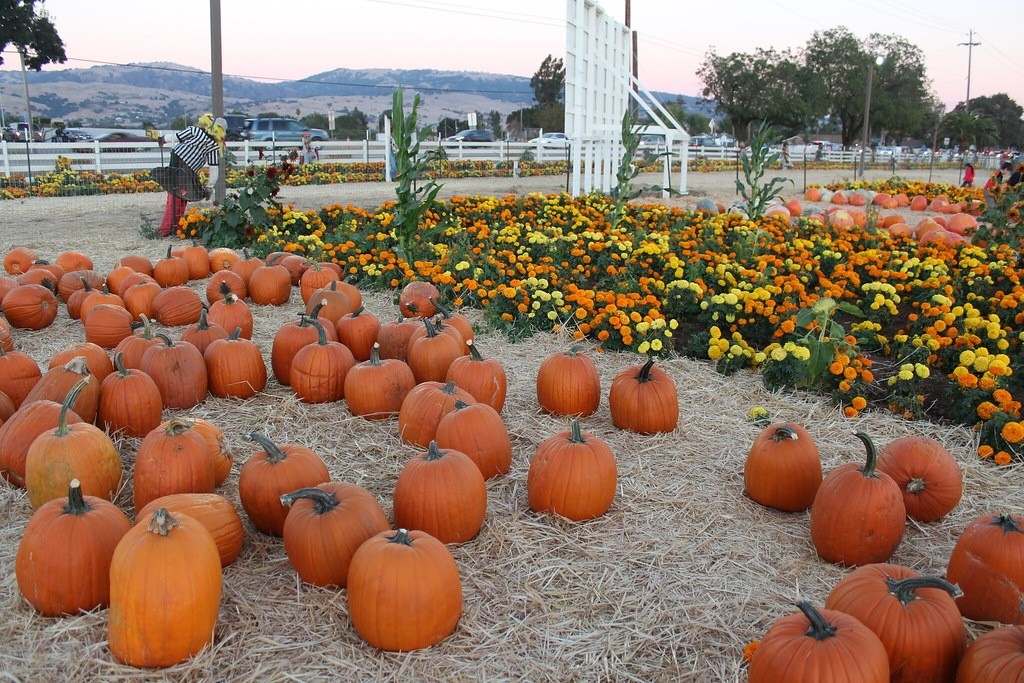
858;56;883;176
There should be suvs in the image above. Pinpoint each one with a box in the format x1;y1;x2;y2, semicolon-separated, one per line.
240;117;329;152
221;113;248;150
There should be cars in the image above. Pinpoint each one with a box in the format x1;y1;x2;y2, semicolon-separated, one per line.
446;129;494;149
689;135;1020;160
528;133;571;150
77;131;152;152
52;129;93;141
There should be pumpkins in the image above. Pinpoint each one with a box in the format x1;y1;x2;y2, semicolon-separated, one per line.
0;239;680;668
697;186;1024;254
743;422;1024;683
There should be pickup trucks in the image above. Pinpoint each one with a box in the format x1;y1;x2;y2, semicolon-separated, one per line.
15;122;45;142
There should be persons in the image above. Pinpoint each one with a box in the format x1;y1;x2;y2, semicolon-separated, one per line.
961;151;1012;209
158;114;227;238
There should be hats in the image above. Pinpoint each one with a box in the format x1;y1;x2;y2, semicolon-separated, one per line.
210;117;227;133
